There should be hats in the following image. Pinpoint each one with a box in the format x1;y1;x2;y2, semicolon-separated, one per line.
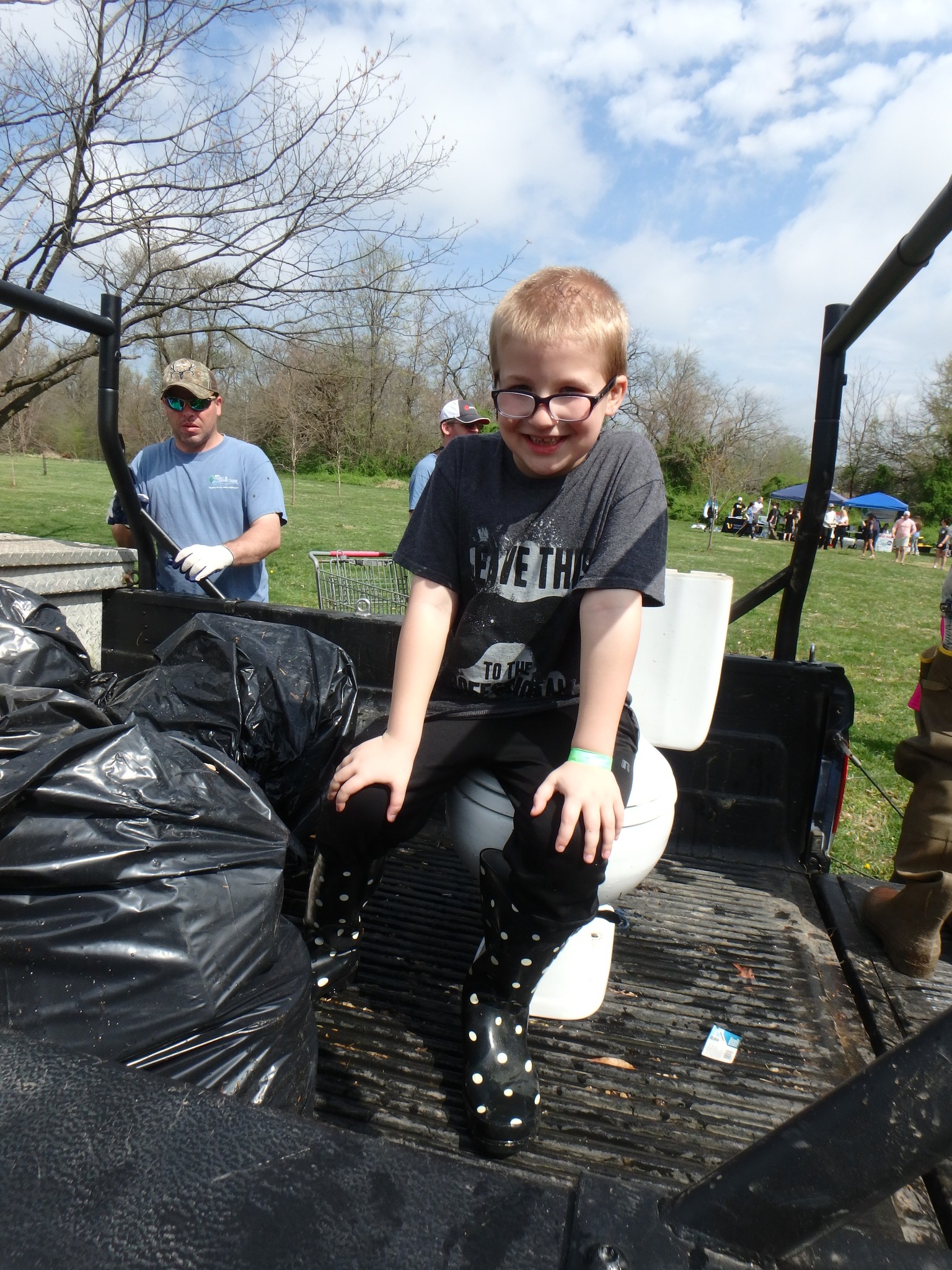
903;511;910;515
440;398;490;425
828;503;835;507
738;497;742;501
160;358;219;400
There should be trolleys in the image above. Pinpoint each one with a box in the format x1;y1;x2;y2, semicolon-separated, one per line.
308;550;413;617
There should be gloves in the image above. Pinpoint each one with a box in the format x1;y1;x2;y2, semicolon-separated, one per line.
167;544;234;583
105;491;149;525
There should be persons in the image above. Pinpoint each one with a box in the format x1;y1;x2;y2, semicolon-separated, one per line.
764;502;778;540
306;265;668;1151
859;513;889;558
892;511;922;565
933;517;951;569
703;495;764;540
863;563;952;977
817;503;849;550
949;524;952;557
106;357;287;603
409;398;490;519
783;508;801;542
769;503;785;539
858;520;868;532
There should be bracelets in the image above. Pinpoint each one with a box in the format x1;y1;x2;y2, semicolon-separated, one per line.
941;542;943;545
567;748;613;770
743;510;744;512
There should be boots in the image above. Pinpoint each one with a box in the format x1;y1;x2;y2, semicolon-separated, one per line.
453;844;598;1155
865;644;952;978
297;855;385;1002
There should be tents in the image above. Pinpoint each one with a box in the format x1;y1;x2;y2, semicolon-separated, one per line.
766;483;846;517
841;492;909;511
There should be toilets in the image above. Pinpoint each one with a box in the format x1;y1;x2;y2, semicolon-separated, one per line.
456;565;738;1020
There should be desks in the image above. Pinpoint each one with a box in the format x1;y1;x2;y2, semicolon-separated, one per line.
721;516;746;535
846;530;862;538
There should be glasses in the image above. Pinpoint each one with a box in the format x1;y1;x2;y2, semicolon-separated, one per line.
491;374;615;422
164;397;215;411
452;421;483;430
